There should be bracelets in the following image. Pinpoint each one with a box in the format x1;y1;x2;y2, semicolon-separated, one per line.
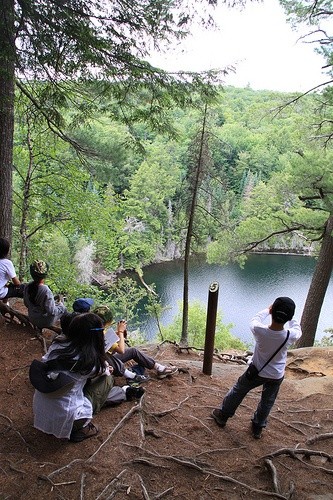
118;330;124;333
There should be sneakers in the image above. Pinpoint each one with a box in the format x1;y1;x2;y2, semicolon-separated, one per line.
157;366;179;379
252;421;262;439
211;409;227;425
133;374;150;383
123;386;144;398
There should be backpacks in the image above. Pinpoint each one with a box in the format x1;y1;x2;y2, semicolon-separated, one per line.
27;354;82;394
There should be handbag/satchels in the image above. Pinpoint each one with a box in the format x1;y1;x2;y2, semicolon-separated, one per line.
248;330;291;378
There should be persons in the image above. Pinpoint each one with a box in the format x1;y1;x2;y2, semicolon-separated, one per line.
211;296;303;440
0;238;179;443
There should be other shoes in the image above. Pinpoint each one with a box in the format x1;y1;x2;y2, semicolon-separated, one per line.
73;427;96;441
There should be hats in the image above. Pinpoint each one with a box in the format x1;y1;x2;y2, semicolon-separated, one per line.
32;259;49;277
74;297;93;310
